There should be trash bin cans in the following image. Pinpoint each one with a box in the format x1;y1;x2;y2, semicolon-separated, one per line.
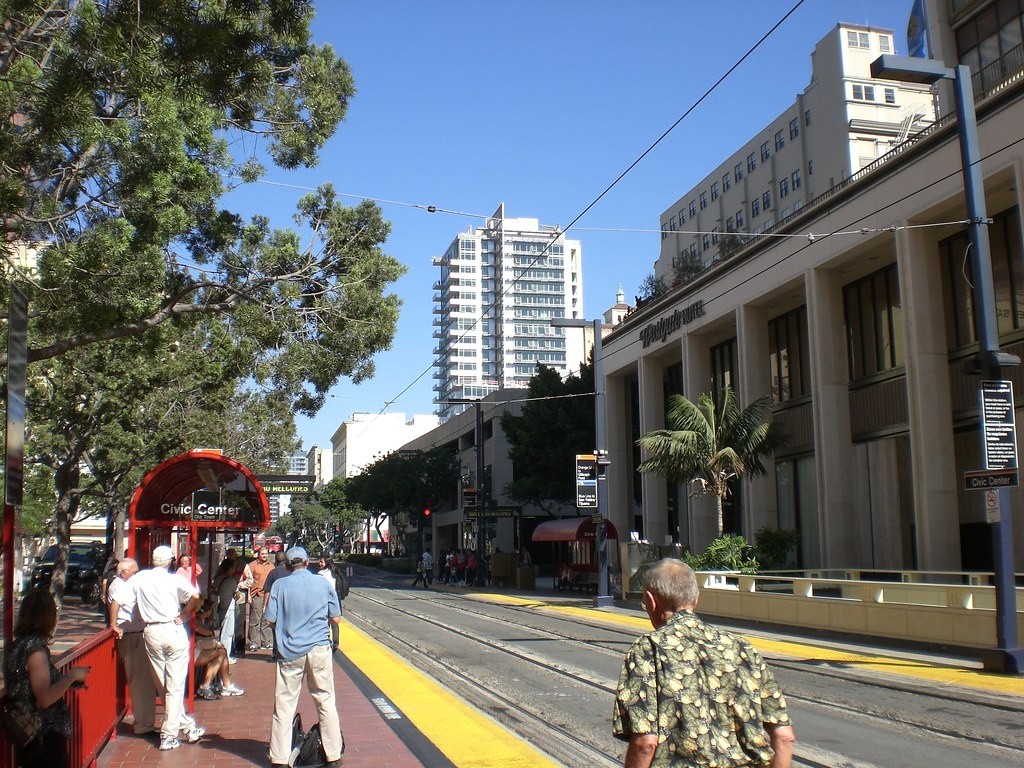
517;563;536;591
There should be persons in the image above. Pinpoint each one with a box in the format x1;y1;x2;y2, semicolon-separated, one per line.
264;547;345;768
318;554;340;652
394;548;400;557
611;558;794;768
557;565;577;591
174;546;293;700
517;548;533;588
0;588;92;768
101;548;128;628
111;546;206;750
412;548;475;590
108;558;160;739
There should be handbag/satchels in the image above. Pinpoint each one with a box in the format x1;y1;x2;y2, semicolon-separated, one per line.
0;693;41;750
417;568;423;572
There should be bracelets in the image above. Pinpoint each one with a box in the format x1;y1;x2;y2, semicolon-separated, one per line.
262;606;267;609
62;675;73;684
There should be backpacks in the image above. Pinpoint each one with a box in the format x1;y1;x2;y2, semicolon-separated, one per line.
331;567;349;600
287;713;345;768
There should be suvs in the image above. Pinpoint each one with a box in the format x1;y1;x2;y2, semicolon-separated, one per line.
30;540;107;604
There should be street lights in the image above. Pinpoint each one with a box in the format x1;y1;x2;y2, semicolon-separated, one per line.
550;317;614;608
447;398;485;587
869;55;1024;674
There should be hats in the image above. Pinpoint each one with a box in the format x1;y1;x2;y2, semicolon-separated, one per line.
284;546;309;563
153;546;174;561
198;599;215;614
276;552;285;561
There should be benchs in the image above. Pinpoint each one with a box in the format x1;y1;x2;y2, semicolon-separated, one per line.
557;583;593;594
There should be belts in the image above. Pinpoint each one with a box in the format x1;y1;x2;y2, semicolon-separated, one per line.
146;621;170;626
123;630;143;634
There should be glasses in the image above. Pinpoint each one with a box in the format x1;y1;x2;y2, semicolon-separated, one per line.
55;606;60;609
125;569;138;575
639;601;649;611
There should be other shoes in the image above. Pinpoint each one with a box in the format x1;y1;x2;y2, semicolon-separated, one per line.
209;682;234;692
333;642;338;652
261;643;273;649
228;658;236;665
134;729;160;738
271;763;284;768
328;759;344;768
249;645;257;652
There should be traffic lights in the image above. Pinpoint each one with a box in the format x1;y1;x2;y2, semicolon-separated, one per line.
423;508;431;517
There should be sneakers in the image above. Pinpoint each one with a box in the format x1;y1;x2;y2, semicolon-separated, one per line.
186;728;205;744
158;738;179;750
220;684;245;696
197;685;218;700
